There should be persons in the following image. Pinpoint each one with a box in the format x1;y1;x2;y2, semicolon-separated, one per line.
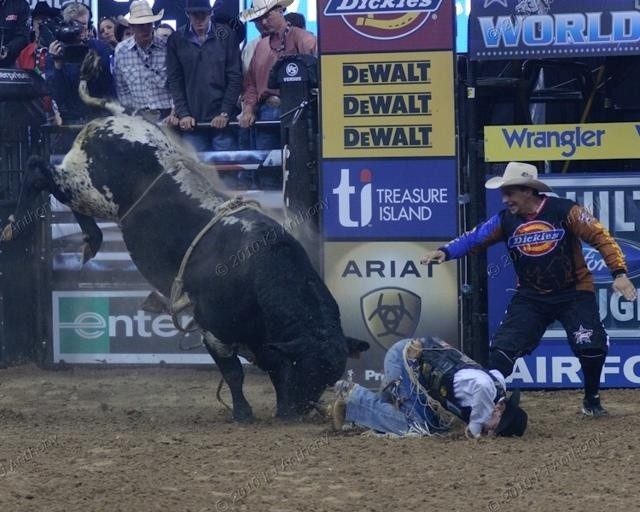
329;336;528;440
1;1;318;192
420;161;638;418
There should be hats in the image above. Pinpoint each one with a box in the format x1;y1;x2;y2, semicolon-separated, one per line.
494;390;527;436
240;0;293;22
484;162;553;192
118;0;164;26
30;2;58;15
176;0;222;12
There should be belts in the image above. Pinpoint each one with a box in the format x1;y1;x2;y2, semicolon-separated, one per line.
266;96;280;108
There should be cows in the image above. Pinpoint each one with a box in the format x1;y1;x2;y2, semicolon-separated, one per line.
0;48;371;422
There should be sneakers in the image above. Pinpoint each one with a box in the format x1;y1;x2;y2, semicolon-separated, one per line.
582;398;608;416
330;380;354;432
